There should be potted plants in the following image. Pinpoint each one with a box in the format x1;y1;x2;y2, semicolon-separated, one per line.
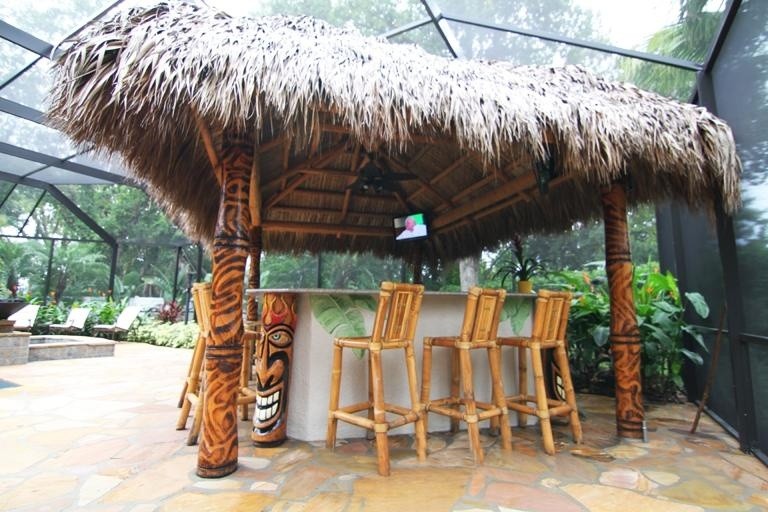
487;241;555;294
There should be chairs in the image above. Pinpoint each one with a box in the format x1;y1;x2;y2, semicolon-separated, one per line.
328;283;425;475
491;289;582;455
175;282;261;447
4;303;142;340
422;290;511;465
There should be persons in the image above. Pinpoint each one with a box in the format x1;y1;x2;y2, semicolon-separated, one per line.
396;217;427;240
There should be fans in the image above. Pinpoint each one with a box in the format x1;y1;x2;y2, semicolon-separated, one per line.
340;152;418;197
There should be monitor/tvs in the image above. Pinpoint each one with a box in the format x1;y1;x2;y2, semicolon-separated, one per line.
392;212;431;241
